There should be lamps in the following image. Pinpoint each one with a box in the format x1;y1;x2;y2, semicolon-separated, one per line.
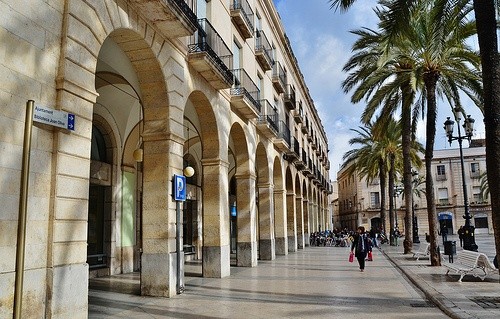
184;127;195;177
134;101;143;162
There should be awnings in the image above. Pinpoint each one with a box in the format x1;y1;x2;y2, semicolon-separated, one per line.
438;211;452;220
474;213;488;218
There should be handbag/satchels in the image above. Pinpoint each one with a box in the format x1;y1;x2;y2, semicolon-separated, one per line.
349;253;354;262
368;251;372;261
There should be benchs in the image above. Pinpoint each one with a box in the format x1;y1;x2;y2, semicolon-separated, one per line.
410;242;430;262
442;249;499;285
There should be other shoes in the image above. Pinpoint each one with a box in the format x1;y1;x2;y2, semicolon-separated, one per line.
360;269;364;272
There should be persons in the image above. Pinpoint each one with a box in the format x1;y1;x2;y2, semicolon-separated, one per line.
351;227;371;272
390;226;404;246
440;224;448;245
425;243;431;262
310;225;389;253
458;226;464;247
